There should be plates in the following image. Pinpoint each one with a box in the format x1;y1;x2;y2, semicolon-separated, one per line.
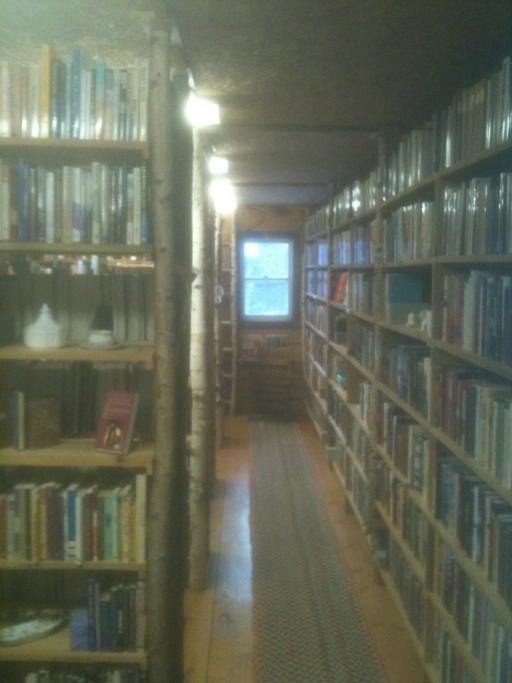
81;344;121;351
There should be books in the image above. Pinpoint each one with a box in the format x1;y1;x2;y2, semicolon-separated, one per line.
333;270;375;317
1;253;157;346
302;295;328;436
0;570;138;653
0;471;152;564
304;204;327;237
0;41;150;143
379;54;512;263
23;665;140;683
328;308;374;550
0;361;155;455
305;267;327;299
0;155;154;247
330;217;377;266
305;236;328;266
328;167;380;230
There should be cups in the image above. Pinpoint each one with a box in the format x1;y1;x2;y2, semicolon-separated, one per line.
89;329;114;346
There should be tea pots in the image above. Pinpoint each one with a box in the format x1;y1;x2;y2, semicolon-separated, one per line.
24;303;63;349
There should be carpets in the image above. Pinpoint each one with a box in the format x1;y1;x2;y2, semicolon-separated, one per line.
247;412;393;682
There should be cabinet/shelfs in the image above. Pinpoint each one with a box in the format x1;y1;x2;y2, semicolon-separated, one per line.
300;196;332;444
383;56;512;681
326;156;381;583
1;29;189;678
